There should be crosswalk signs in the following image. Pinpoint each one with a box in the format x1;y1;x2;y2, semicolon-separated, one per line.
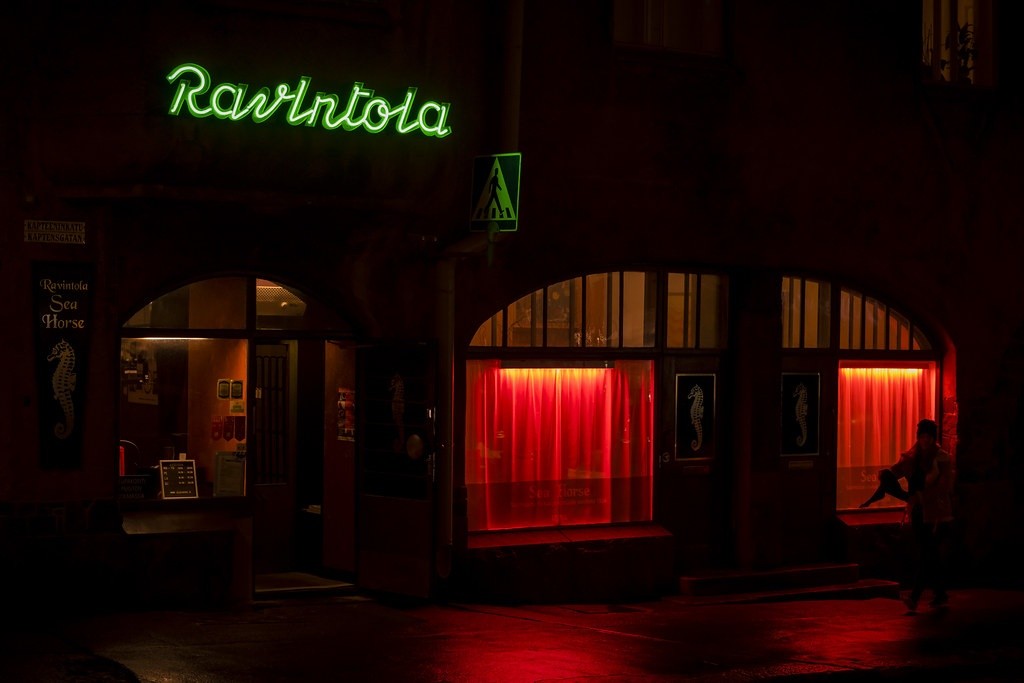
468;152;521;232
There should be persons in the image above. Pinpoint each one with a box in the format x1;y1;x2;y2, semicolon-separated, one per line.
889;420;953;610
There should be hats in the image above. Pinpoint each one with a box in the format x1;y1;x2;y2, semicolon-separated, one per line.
917;419;937;442
